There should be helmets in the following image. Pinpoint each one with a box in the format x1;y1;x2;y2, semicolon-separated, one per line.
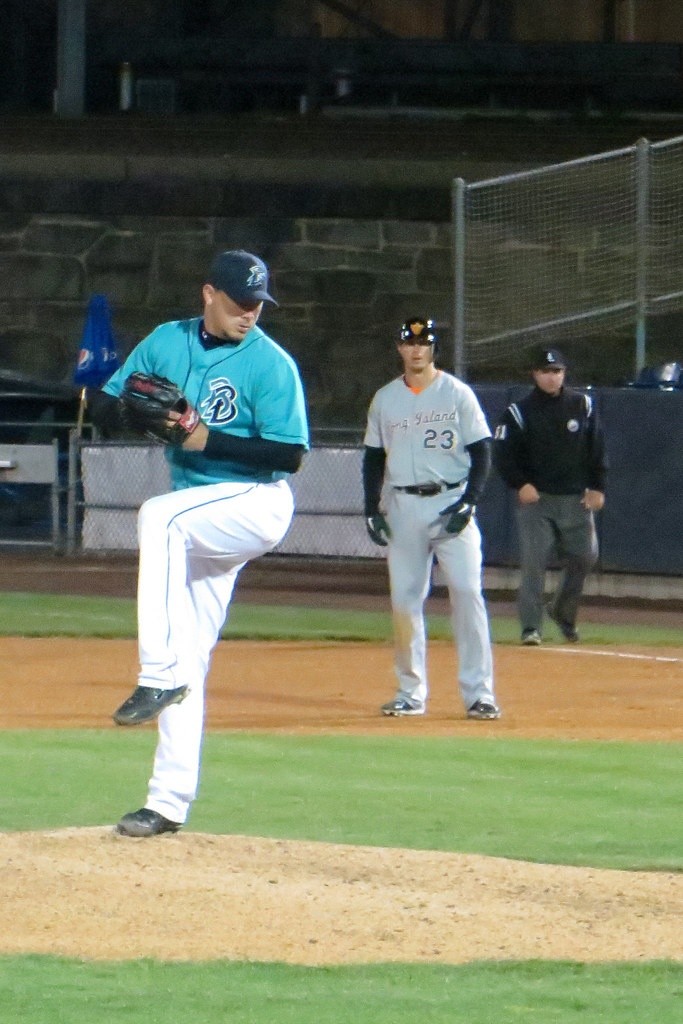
398;318;437;347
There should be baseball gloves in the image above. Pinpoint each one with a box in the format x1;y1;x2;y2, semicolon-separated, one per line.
118;371;200;447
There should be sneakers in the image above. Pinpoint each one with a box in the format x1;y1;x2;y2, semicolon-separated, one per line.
117;808;182;838
521;628;543;645
469;701;501;720
547;601;579;643
112;683;191;728
381;700;424;717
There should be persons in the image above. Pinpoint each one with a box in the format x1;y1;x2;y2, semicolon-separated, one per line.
361;314;505;721
95;250;312;838
498;346;610;643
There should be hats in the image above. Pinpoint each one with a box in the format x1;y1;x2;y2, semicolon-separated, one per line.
204;249;280;304
532;348;568;371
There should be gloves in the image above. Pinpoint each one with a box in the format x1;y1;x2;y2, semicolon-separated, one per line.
439;501;477;534
364;514;393;548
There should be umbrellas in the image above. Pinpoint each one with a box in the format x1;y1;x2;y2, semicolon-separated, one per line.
74;290;121;387
